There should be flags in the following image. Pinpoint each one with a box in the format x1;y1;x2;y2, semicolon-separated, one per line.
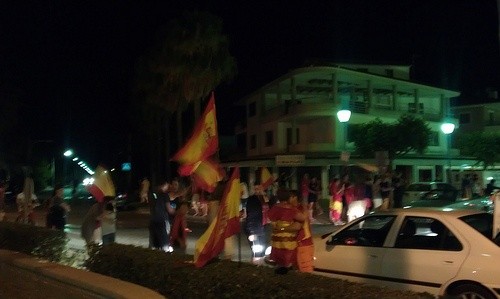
87;165;115;202
172;96;218;163
260;167;273;187
192;167;241;269
178;157;225;194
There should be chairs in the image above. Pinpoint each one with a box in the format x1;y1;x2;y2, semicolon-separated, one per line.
431;221;449;240
404;220;417;237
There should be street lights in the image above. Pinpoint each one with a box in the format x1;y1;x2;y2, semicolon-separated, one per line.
441;123;455;185
337;109;352;186
51;149;95;189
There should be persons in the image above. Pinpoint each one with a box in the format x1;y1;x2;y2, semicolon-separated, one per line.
300;173;324;221
371;171;405;209
14;190;38;225
329;177;371;227
461;173;500;200
148;177;186;254
49;183;72;231
243;183;315;273
79;195;117;244
96;203;117;244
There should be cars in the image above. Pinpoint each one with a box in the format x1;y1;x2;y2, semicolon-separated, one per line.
83;177;128;207
401;182;500;212
265;207;500;299
